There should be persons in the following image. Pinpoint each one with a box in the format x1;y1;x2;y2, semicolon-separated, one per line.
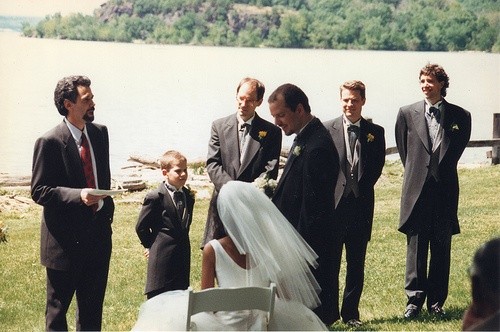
463;237;500;332
321;81;385;327
131;181;329;331
269;83;341;281
200;78;282;251
394;63;471;321
135;150;195;300
31;75;116;332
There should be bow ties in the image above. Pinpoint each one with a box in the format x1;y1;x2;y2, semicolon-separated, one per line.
347;126;359;136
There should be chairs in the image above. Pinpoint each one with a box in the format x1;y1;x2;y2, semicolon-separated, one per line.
186;283;276;331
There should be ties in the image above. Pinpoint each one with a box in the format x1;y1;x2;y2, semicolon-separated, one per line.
166;183;185;220
240;123;251;170
285;135;297;168
429;106;436;119
80;132;99;217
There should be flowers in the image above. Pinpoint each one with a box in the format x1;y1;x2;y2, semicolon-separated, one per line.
251;177;279;197
294;145;302;156
367;134;377;143
452;122;461;131
258;130;267;138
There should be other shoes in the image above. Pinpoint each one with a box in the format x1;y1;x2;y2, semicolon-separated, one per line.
429;307;450;321
404;309;420;320
346;319;363;328
